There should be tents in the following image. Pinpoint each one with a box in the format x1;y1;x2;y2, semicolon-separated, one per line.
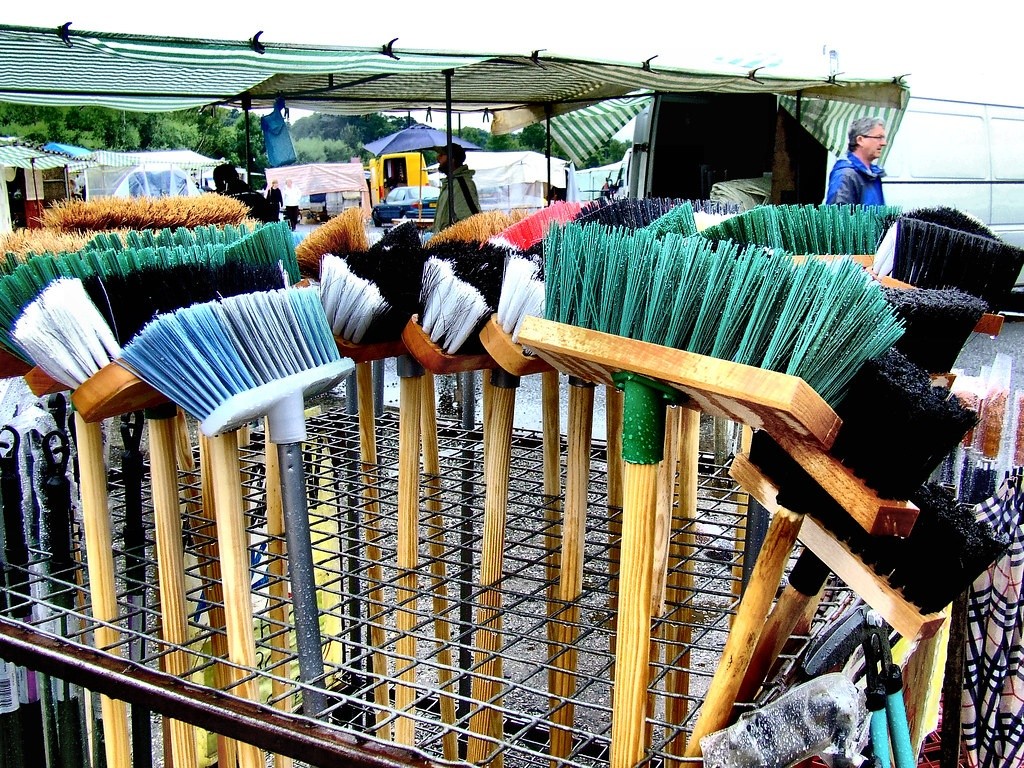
0;22;909;226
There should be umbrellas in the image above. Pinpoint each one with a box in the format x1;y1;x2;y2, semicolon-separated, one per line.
362;123;483;220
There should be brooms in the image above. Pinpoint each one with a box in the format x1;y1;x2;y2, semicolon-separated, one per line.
0;191;1024;768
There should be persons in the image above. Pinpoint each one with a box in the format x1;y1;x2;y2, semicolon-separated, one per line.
826;118;886;217
208;161;265;223
70;179;82;201
431;143;483;235
282;178;301;232
266;180;283;224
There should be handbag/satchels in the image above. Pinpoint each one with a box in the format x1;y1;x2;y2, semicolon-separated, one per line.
261;109;296;168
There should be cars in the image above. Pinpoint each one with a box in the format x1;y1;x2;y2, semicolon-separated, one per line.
372;185;443;230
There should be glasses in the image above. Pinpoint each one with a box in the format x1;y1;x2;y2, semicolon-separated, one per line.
861;135;886;141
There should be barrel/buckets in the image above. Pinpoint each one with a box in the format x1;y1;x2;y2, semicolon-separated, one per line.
24;200;44;229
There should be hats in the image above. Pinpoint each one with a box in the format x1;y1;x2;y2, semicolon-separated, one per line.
433;143;466;162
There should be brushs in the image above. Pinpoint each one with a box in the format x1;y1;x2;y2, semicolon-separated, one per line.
2;181;1024;768
